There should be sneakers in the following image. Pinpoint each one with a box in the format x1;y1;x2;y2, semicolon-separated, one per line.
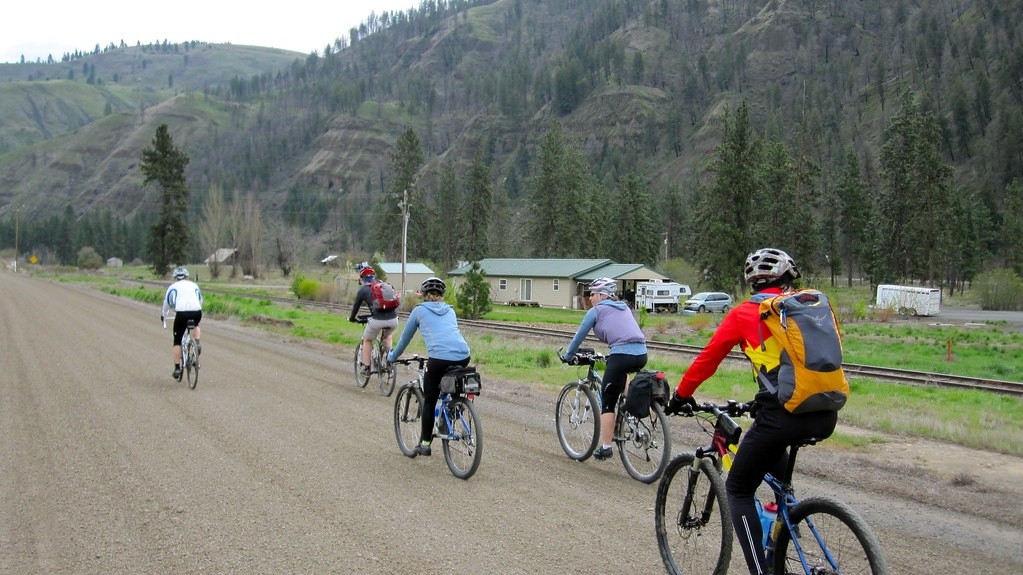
415;442;432;456
442;404;463;418
360;368;371;378
593;445;614;457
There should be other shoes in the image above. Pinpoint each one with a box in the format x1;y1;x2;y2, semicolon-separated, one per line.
193;345;201;356
172;370;180;377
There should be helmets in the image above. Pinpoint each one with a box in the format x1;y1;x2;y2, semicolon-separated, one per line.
360;266;375;278
744;248;800;293
421;277;447;296
589;278;618;298
173;267;189;279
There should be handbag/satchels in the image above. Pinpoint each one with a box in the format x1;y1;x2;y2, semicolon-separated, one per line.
625;369;671;418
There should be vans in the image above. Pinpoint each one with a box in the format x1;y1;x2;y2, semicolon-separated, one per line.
684;292;734;313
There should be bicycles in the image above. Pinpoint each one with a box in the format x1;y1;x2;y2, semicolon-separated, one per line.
386;349;483;480
162;317;198;390
655;397;887;575
346;314;397;397
555;347;672;484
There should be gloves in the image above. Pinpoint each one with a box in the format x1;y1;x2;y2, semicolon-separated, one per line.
665;389;701;418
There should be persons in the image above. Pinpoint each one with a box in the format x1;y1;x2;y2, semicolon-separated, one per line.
668;248;838;575
386;278;471;456
161;267;203;377
348;266;398;377
562;277;648;458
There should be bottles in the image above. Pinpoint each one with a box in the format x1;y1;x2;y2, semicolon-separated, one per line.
372;344;378;358
753;495;778;551
435;399;443;423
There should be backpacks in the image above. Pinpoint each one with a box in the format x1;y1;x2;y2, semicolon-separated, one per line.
746;288;849;414
365;280;401;311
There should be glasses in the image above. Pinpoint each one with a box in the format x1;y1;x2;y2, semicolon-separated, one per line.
589;291;602;297
